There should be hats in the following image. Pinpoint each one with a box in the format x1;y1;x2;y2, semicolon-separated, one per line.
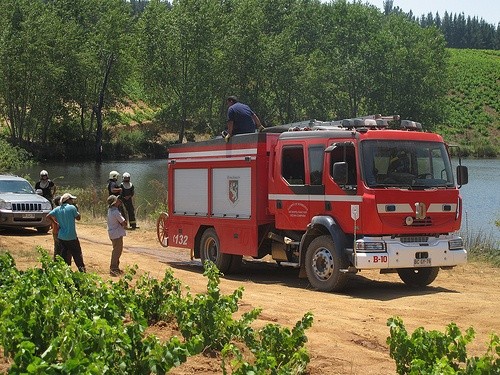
60;193;77;203
53;195;62;204
107;195;121;208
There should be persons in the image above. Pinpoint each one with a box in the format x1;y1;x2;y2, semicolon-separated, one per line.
107;170;140;230
35;170;57;210
107;195;128;277
44;193;86;274
224;96;264;143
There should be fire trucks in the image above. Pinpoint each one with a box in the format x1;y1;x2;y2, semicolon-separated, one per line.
155;113;468;293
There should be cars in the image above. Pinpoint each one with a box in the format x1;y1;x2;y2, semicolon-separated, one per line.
0;174;53;233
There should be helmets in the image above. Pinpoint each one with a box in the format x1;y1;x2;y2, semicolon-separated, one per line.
122;171;131;181
108;170;119;181
39;169;49;180
